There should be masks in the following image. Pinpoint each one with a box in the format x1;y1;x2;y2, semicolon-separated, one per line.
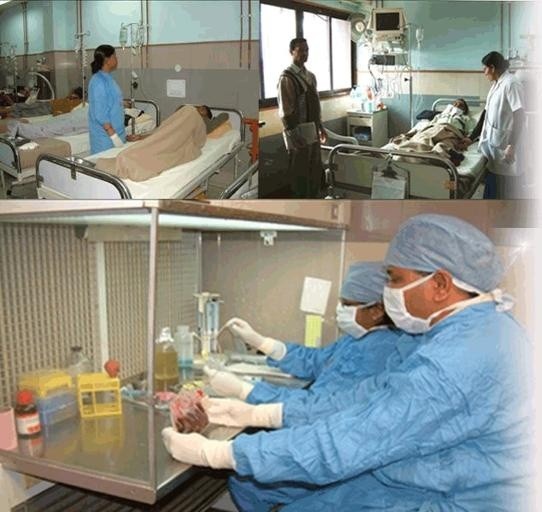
336;304;367;339
382;274;434;335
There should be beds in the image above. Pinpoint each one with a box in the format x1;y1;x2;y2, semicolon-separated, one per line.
322;98;492;200
1;93;246;200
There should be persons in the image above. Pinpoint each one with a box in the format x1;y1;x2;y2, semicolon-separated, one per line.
456;50;527;200
0;85;135;141
127;103;230;142
89;45;128;155
275;38;331;199
392;99;472;153
159;211;534;512
198;259;403;512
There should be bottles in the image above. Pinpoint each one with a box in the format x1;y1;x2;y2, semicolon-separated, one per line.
174;325;194;370
64;345;92;374
119;28;127;46
14;391;42;437
155;325;180;380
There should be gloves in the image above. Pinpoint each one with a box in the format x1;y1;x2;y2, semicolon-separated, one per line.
204;364;254;400
162;427;237;471
204;398;284;431
110;133;122;148
222;318;287;362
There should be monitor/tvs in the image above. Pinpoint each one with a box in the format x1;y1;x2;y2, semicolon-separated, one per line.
372;8;404;34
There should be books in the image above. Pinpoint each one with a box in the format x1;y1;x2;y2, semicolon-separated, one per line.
282;121;317;151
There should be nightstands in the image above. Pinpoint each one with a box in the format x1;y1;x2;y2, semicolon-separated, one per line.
346;108;389;149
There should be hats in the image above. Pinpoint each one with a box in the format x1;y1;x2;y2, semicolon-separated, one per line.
340;260;386;304
382;214;501;293
